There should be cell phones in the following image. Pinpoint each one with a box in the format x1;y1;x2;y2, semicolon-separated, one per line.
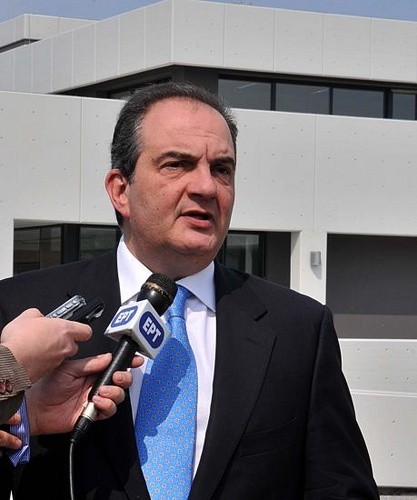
68;298;105;326
45;293;86;321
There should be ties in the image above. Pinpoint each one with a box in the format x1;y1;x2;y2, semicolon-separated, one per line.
134;285;199;500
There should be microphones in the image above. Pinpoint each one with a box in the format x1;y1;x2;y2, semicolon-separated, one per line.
74;273;178;433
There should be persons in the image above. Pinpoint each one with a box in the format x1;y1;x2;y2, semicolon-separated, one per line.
0;82;380;500
1;305;92;401
2;354;145;450
